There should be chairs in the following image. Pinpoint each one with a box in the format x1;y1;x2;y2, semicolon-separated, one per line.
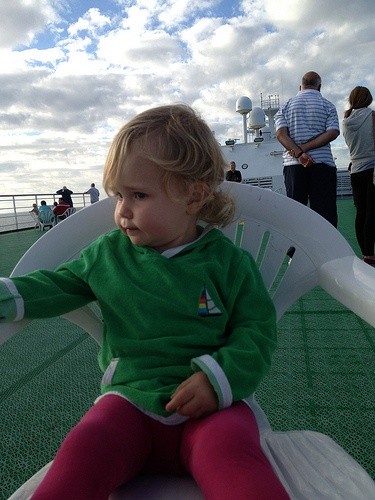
0;180;375;500
